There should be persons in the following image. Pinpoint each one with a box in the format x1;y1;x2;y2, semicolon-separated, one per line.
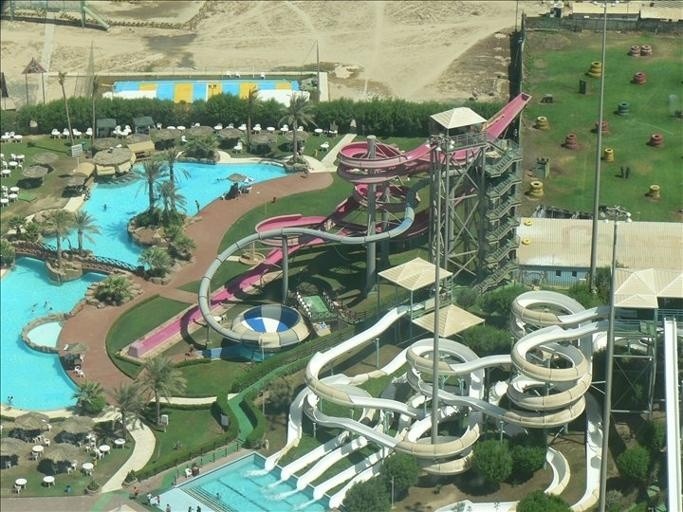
195;200;200;213
134;487;201;512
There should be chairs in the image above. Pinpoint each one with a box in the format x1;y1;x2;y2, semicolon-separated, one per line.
0;152;24;207
0;420;125;493
0;130;21;142
112;125;132;142
154;123;339;152
51;126;93;141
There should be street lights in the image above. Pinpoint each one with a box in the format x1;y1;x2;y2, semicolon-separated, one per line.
573;0;631;294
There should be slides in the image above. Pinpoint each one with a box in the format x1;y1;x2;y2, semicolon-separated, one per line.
265;290;611;511
665;317;680;512
131;93;534;365
197;166;468;362
